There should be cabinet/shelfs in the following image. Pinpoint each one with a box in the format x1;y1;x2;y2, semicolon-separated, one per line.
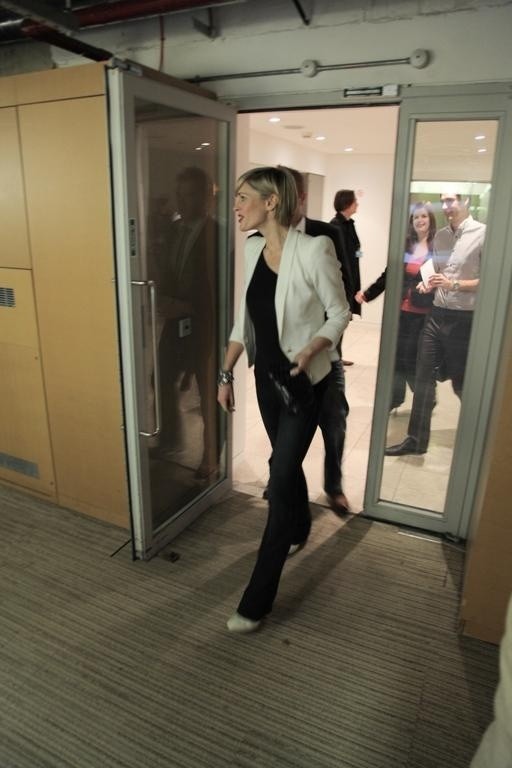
1;60;220;533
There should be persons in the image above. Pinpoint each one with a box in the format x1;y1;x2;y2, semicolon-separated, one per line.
217;166;350;638
384;194;488;456
247;167;351;560
362;201;438;413
330;190;362;317
152;165;224;483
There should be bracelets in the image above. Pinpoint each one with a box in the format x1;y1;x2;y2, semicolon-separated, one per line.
216;367;237;390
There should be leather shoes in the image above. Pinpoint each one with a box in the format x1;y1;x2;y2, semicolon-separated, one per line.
384;437;430;457
324;492;353;514
224;607;271;636
